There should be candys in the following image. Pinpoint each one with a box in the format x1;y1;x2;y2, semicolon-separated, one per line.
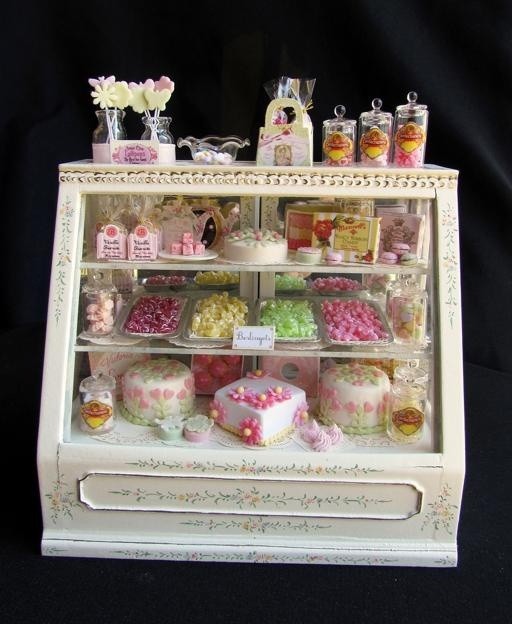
89;75;174;142
81;392;113;433
110;269;393;293
322;123;426;168
192;149;232;165
85;294;427;341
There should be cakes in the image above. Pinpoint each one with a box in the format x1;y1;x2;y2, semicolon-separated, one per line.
224;226;287;263
116;355;391;451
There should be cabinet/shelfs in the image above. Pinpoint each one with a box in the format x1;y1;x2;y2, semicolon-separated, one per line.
37;157;467;568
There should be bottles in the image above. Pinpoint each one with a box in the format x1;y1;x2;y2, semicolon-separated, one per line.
385;359;430;444
77;368;119;436
357;99;392;167
391;89;430;168
384;274;430;350
94;108;126;145
322;104;358;167
81;272;118;339
140;116;173;142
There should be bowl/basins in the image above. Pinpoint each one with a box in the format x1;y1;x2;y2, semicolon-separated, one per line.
176;132;252;164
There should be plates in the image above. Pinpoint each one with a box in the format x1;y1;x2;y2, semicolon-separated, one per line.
157;249;218;262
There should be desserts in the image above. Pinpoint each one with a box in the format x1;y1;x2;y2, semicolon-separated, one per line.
285;208;417;266
96;205;227;263
